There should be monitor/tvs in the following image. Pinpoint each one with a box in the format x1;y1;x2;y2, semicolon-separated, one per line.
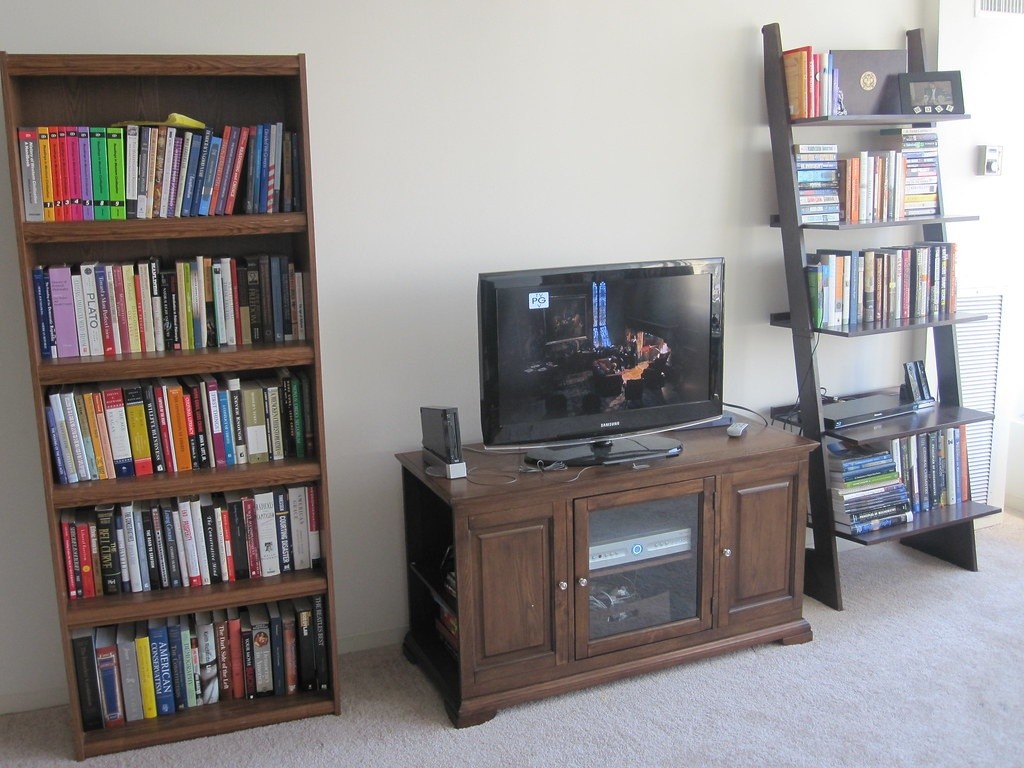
478;257;725;449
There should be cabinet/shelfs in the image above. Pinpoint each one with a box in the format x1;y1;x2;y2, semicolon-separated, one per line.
0;48;349;756
758;21;1003;620
389;415;816;734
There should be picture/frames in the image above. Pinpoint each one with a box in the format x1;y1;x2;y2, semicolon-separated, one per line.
895;69;968;117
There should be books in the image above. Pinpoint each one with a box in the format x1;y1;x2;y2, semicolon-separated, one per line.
15;112;329;734
781;45;972;538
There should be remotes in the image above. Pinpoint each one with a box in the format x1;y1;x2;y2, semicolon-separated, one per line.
727;422;750;437
